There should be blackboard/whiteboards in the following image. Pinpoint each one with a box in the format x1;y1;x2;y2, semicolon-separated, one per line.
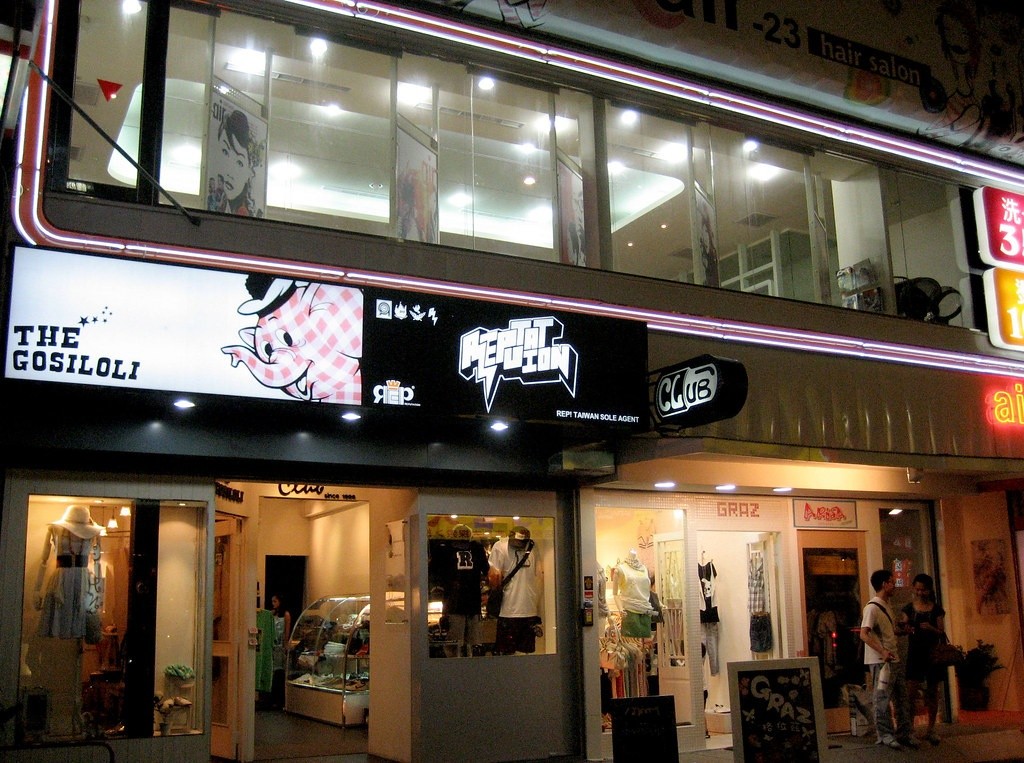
725;657;830;762
611;697;679;763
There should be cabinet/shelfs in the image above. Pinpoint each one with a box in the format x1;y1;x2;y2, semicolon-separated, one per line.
284;594;370;727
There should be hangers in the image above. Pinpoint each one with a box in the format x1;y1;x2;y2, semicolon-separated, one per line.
599;629;650;654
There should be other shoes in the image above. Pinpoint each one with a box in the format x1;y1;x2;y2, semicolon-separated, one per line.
926;728;941;744
894;732;922;748
877;733;902;750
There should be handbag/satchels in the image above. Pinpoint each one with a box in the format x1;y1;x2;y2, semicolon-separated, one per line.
931;632;964;663
486;588;504;617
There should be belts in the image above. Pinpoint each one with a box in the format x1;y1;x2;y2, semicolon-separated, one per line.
57;553;89;567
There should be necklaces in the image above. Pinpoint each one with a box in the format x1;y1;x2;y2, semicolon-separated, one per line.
67;531;85;558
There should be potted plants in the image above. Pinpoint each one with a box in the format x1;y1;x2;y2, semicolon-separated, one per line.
953;639;1008;710
165;663;195;687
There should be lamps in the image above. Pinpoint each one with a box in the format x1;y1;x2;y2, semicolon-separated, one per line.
906;467;925;483
99;506;131;537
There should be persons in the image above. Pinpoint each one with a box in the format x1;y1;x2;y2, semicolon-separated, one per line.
612;550;668;638
270;596;290;720
698;642;712;740
430;524;544;658
33;505;101;641
859;570;922;749
898;573;945;746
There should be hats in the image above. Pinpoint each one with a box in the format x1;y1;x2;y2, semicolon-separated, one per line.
509;525;531;549
46;504;104;539
452;524;472;540
